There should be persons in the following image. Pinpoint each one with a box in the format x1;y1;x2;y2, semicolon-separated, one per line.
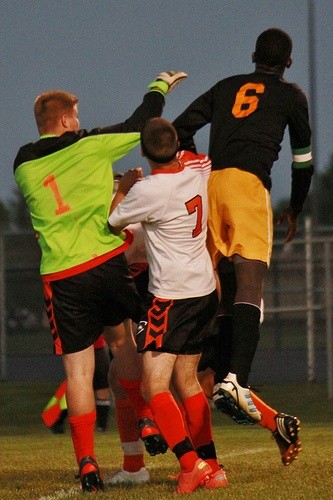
43;223;301;483
107;117;228;495
172;27;314;426
13;71;187;490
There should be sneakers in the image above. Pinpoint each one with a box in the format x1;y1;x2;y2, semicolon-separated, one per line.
171;458;212;495
137;417;167;456
213;372;261;426
271;413;301;467
76;456;101;494
105;465;150;485
198;468;228;489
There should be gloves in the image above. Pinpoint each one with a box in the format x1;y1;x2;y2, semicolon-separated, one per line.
148;70;188;94
